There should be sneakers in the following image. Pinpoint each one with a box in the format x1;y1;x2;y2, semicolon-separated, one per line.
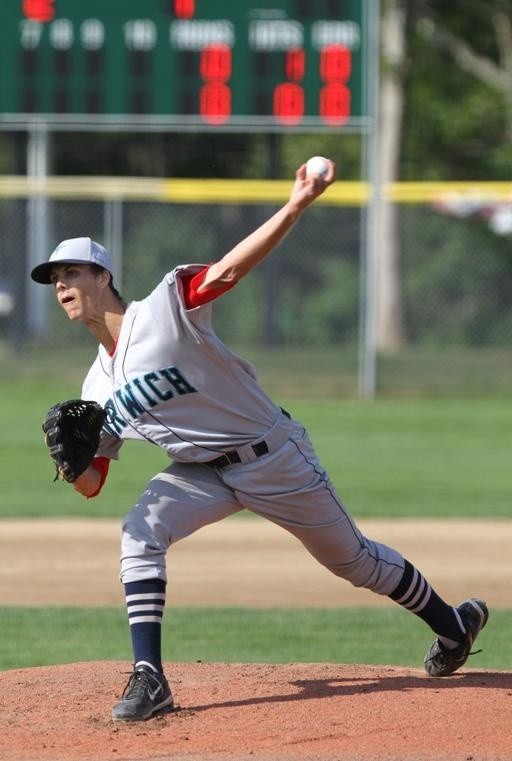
424;597;489;678
111;670;174;721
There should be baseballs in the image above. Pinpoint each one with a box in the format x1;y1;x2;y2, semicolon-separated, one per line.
305;157;330;177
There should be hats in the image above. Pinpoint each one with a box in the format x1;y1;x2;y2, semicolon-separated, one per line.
31;237;113;285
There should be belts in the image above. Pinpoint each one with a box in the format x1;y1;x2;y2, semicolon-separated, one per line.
208;406;292;470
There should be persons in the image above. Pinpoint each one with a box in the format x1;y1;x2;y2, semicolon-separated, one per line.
29;155;491;724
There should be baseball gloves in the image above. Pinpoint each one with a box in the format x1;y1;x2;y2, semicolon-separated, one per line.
42;399;108;484
